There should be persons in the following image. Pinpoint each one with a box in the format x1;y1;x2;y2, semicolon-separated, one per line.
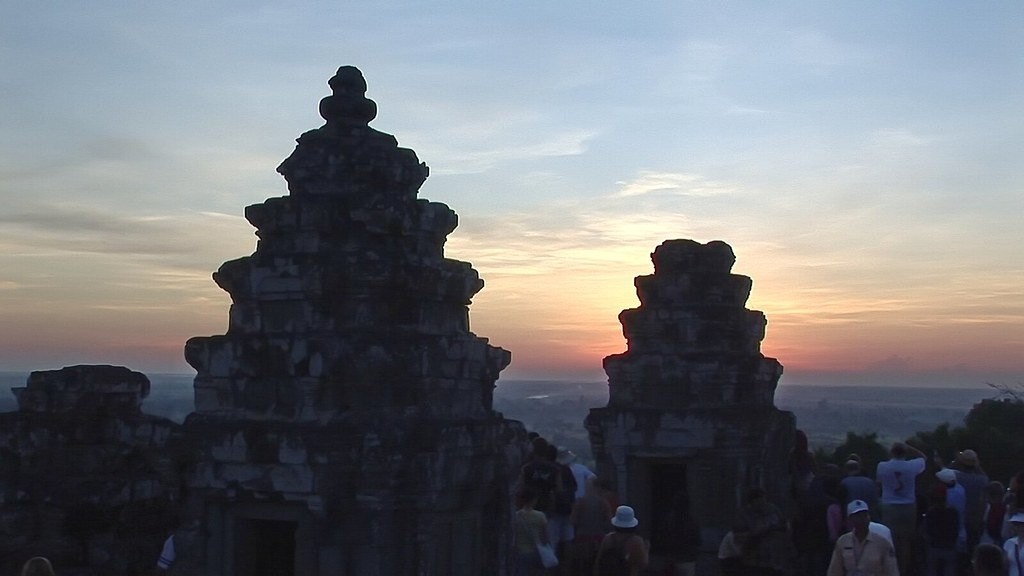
153;515;181;576
514;439;1024;576
24;557;54;576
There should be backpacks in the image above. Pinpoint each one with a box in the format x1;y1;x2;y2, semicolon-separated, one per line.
598;532;634;576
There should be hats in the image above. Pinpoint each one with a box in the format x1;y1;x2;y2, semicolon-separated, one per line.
611;505;639;528
934;482;947;496
847;500;869;516
935;469;957;482
959;450;979;466
1007;512;1024;522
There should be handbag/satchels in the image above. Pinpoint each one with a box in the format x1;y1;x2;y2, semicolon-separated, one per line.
534;542;559;569
